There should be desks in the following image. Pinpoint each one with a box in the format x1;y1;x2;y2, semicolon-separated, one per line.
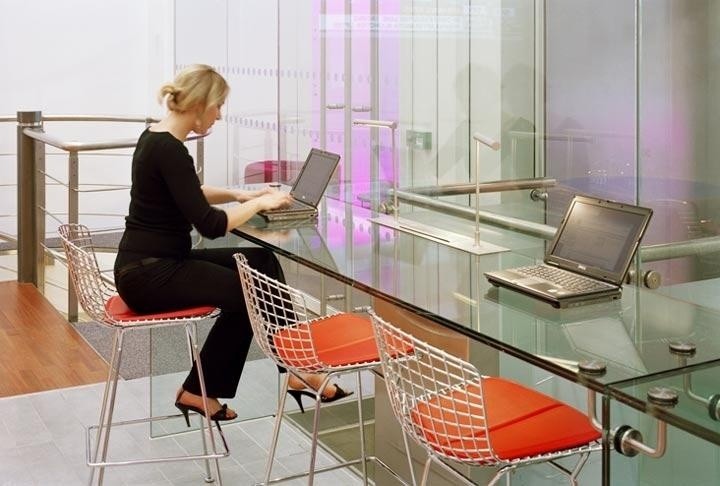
203;182;718;485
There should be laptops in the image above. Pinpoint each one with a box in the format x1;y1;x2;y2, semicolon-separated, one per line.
255;148;341;223
482;194;654;309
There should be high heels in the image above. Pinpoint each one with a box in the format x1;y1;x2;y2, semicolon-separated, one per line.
175;403;237;427
287;383;354;413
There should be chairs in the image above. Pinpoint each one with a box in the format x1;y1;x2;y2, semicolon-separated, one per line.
57;223;230;486
233;252;423;486
368;309;614;485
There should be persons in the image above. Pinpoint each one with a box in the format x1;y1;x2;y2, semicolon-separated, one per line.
113;62;356;428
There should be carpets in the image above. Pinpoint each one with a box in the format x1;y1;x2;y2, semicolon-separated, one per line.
67;312;266;381
